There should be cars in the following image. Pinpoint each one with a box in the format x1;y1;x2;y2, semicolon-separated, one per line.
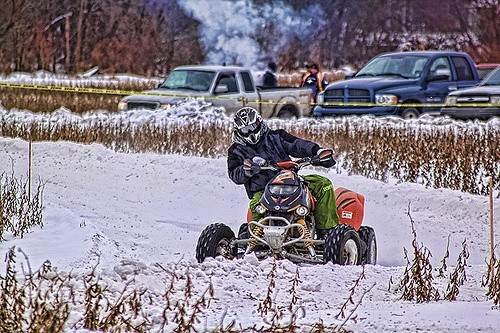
477;64;500;79
440;66;500;121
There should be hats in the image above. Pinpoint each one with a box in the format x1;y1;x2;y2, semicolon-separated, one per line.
306;63;319;70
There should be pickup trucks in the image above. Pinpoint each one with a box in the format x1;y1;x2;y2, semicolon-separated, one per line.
116;65;314;121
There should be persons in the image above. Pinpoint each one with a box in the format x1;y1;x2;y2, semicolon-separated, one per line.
263;62;278;87
227;107;339;250
299;63;329;102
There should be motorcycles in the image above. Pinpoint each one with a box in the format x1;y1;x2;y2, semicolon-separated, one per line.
197;150;379;266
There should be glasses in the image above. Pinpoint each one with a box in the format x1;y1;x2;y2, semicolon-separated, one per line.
237;120;261;137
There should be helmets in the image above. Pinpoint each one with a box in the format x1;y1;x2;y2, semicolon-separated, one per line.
232;107;267;148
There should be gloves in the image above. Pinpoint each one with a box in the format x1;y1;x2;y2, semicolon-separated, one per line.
244;156;267;176
316;149;336;167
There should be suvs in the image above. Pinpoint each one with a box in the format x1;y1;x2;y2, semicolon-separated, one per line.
313;50;483;120
